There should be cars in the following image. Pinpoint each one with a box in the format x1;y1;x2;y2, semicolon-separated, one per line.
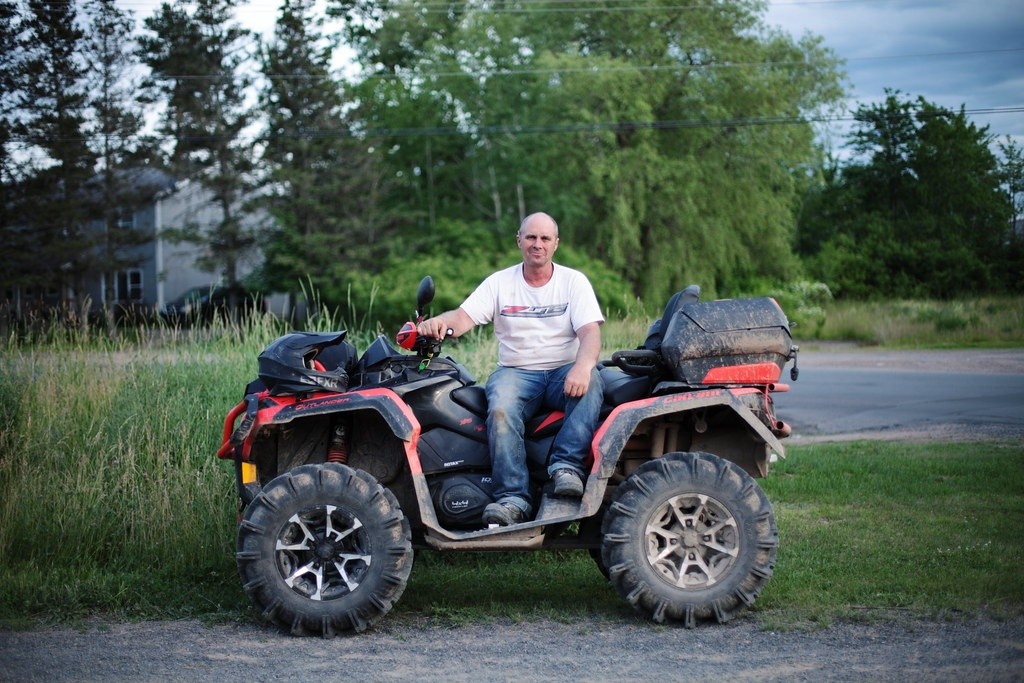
157;282;240;330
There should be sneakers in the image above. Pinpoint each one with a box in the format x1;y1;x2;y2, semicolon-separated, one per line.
553;469;584;497
482;503;524;527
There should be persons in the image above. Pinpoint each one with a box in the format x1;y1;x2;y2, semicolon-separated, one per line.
428;210;605;532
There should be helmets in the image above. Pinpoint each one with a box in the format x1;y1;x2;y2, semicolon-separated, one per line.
256;330;349;394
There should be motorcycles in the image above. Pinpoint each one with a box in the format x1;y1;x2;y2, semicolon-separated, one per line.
216;262;802;637
